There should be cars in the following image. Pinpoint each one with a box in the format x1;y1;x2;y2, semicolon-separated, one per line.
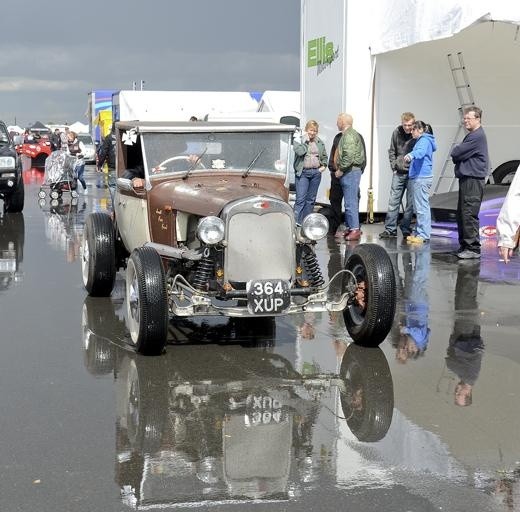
73;133;100;164
81;118;397;356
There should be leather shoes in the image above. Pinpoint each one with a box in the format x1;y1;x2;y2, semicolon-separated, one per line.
346;231;361;241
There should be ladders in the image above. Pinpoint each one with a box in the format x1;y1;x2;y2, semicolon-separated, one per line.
432;52;495;195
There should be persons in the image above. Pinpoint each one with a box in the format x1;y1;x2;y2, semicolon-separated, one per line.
292;120;328;229
7;124;90;197
495;164;520;265
120;133;202;188
402;120;439;242
95;120;120;213
444;258;486;408
379;112;417;239
327;236;359;356
450;107;488;260
399;241;433;359
290;233;323;339
326;112;367;236
333;114;366;241
382;237;410;364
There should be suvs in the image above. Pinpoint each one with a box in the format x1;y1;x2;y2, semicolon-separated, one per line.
0;120;24;212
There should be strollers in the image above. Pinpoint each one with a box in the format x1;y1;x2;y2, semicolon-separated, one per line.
38;150;79;200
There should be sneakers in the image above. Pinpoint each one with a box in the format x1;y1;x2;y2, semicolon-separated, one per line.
451;246;461;256
457;249;480;259
407;235;426;243
83;186;88;195
379;231;397;238
403;233;410;238
